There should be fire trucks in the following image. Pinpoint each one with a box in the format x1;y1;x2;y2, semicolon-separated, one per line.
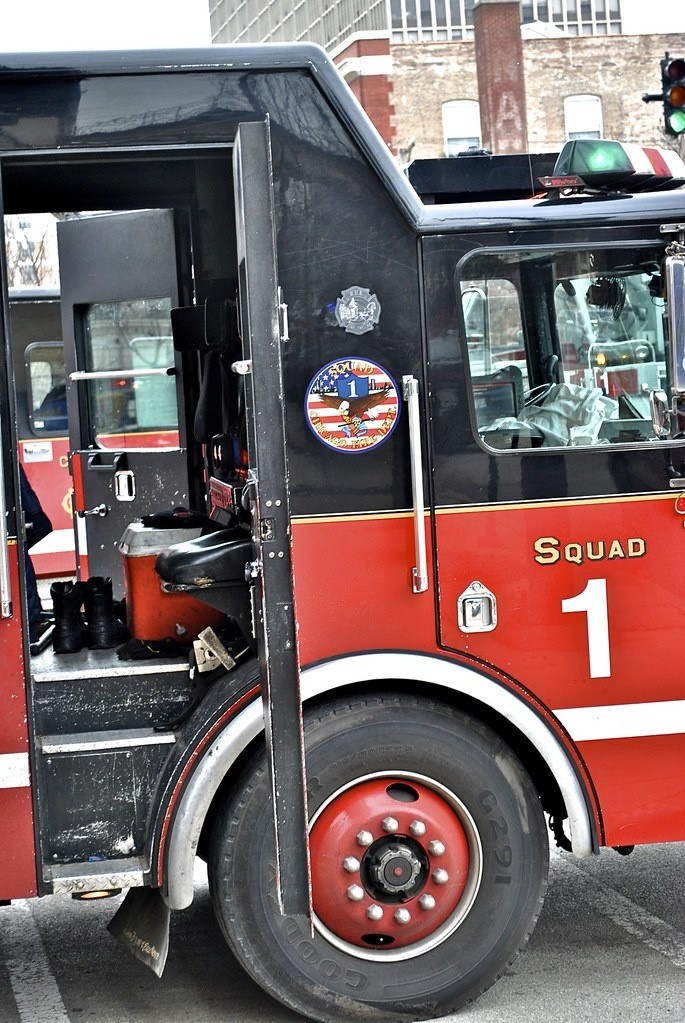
0;43;684;1023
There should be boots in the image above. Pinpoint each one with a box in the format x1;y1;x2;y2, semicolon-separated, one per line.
81;575;126;648
51;581;86;653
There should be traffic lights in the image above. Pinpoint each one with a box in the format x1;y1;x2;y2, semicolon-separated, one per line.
660;57;685;134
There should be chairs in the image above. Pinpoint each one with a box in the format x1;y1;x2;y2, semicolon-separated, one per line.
470;366;533;450
